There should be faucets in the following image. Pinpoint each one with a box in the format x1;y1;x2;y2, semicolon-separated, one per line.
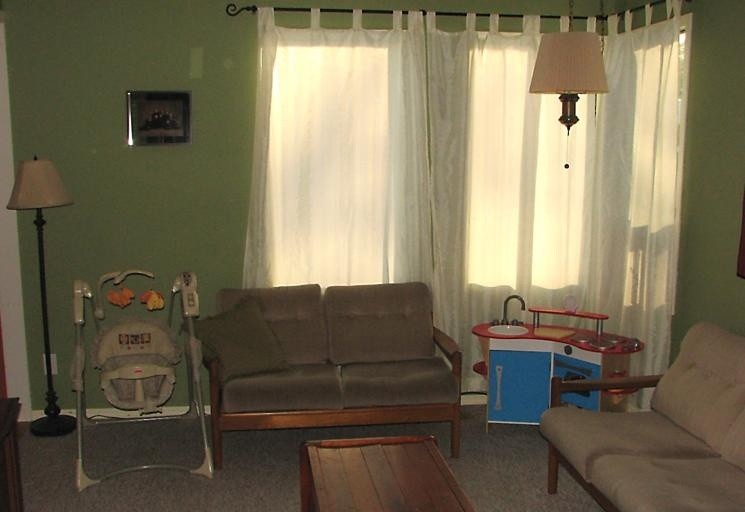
501;293;526;326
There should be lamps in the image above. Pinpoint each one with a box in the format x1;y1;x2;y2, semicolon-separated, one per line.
529;0;609;136
5;154;77;436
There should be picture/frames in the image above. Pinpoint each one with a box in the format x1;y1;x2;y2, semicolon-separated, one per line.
126;90;190;144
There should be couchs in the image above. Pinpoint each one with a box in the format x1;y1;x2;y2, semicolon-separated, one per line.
186;327;462;471
539;322;745;511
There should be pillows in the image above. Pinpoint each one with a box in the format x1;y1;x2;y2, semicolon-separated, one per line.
325;282;435;365
218;284;329;366
650;322;744;458
179;295;288;383
719;408;745;472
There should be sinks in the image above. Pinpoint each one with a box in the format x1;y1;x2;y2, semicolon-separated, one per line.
489;325;529;337
